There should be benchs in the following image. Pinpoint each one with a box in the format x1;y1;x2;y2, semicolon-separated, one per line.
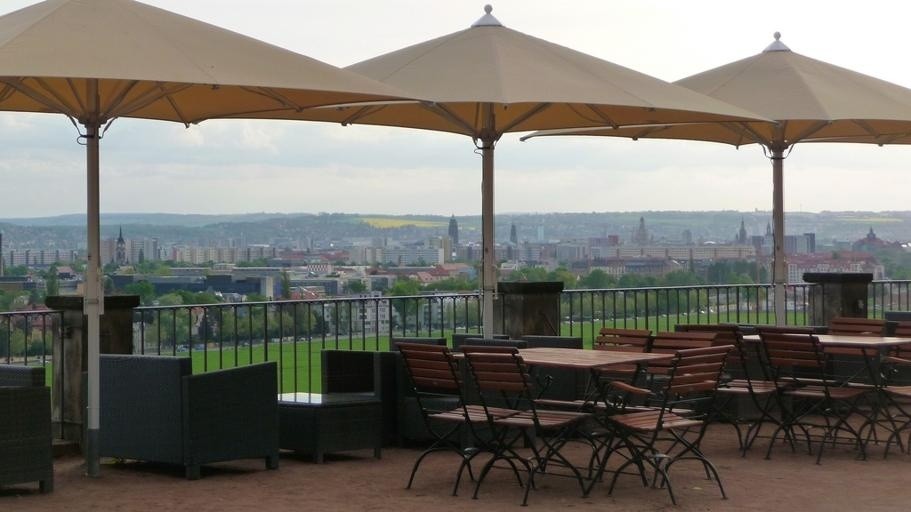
389;311;911;506
320;348;403;447
99;356;278;476
2;365;57;492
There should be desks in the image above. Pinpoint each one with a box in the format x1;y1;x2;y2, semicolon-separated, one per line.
276;390;385;463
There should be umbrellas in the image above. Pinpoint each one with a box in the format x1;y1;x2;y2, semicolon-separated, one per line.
517;29;911;325
216;4;780;339
0;0;420;478
